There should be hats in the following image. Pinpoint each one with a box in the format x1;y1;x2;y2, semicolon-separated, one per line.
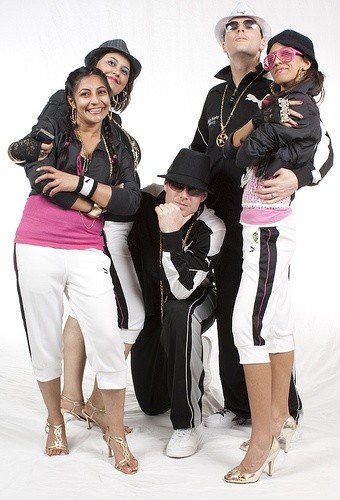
214;3;274;48
156;147;217;195
267;29;319;67
84;39;142;81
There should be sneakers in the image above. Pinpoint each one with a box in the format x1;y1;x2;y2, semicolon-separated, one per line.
206;408;250;428
165;423;205;457
201;336;212;391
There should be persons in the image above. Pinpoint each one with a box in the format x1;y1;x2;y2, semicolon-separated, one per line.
224;30;326;484
189;3;334;429
127;148;226;458
13;66;141;475
8;39;142;435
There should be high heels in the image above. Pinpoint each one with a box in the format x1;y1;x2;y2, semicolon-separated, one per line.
43;416;69;457
103;425;140;475
223;433;280;483
59;393;85;423
239;415;296;453
81;398;134;437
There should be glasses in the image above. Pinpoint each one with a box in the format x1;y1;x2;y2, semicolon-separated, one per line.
262;47;303;70
166;179;204;197
223;19;258;32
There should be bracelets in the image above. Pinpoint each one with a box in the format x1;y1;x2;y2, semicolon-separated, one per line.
75;175;97;198
85;203;102;218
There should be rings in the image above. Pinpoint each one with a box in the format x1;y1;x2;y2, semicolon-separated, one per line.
42;150;43;153
271;194;274;198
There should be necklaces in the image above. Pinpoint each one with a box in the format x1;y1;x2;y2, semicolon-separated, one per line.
216;69;264;147
76;129;91;156
